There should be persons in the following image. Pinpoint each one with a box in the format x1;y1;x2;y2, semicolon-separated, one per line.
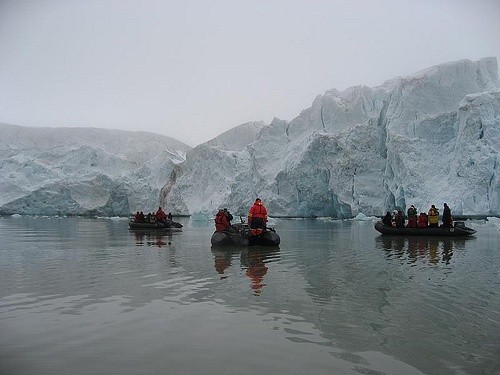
134;206;173;223
383;202;454;228
246;198;268;236
214;207;237;233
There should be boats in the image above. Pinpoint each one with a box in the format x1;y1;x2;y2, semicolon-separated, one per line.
128;216;184;229
210;216;280;247
374;221;477;237
